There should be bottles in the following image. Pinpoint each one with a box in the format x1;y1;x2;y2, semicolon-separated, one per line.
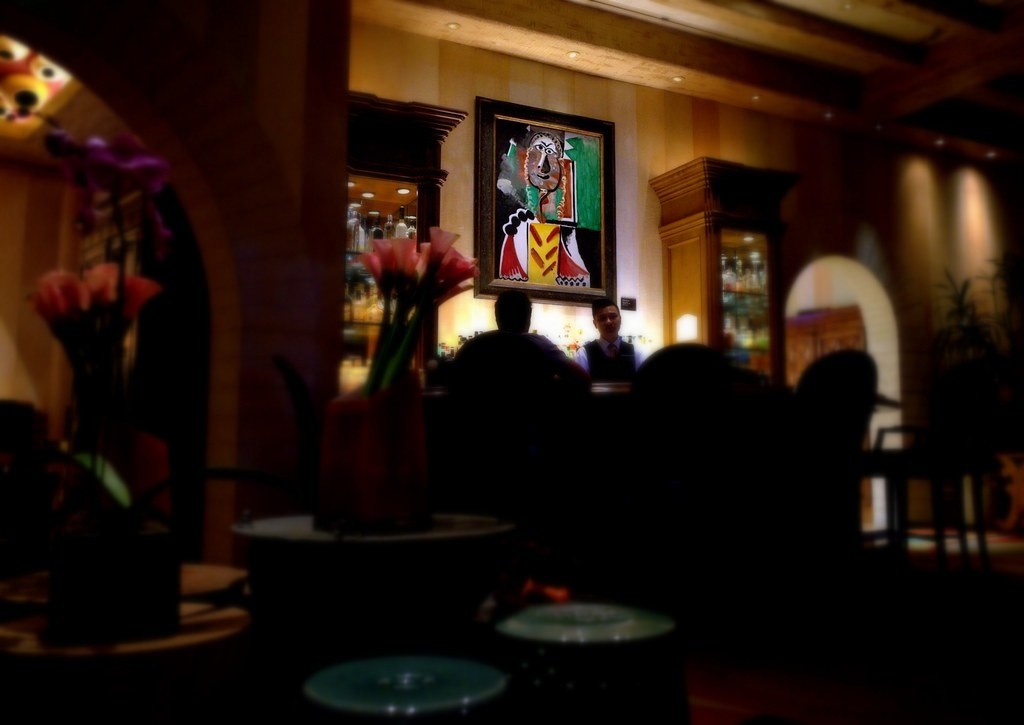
345;280;382;323
721;255;768;294
724;317;772;376
347;202;417;255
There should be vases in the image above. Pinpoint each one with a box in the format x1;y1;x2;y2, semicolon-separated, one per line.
313;368;424;533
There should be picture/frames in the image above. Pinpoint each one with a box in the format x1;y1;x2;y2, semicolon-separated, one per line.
473;95;618;309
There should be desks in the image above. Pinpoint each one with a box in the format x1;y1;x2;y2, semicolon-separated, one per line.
230;514;517;725
0;598;256;725
0;560;257;605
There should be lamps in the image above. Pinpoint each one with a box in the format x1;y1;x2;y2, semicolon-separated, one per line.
0;35;76;135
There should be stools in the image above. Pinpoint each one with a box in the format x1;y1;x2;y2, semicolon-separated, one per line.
301;653;508;721
493;602;693;725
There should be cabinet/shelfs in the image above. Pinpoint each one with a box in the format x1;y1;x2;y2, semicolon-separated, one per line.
648;156;807;386
340;85;468;404
789;305;866;388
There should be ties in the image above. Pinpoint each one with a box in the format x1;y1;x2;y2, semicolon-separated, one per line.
608;343;618;361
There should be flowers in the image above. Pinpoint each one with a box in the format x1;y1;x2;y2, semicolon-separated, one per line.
348;226;479;396
20;261;164;514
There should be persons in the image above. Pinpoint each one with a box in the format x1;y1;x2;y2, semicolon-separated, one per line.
575;299;641;382
450;291;591;398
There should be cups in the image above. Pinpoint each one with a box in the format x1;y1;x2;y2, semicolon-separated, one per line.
303;656;507;725
495;604;692;725
